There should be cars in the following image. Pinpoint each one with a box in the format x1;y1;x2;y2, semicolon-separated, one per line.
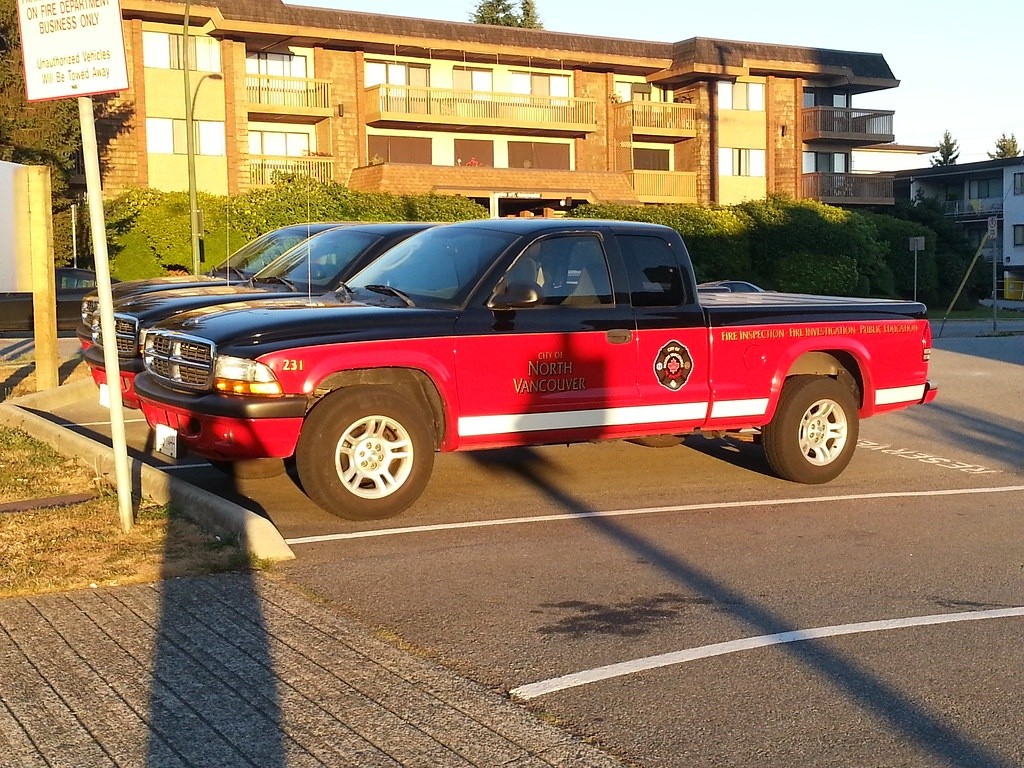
0;268;122;331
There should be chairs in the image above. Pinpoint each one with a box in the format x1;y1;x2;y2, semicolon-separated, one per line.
506;256;537;287
66;277;75;289
559;264;613;305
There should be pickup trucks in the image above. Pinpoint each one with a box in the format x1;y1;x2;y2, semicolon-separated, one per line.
76;220;585;410
136;218;942;522
